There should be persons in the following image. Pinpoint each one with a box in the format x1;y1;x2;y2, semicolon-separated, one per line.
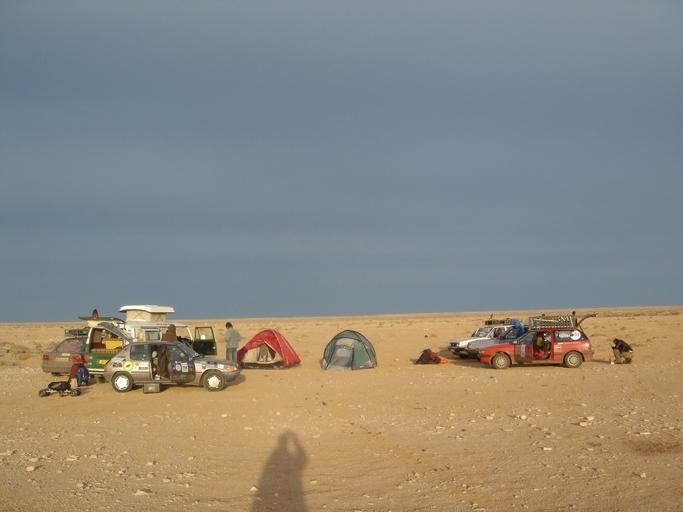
611;339;634;365
223;322;241;364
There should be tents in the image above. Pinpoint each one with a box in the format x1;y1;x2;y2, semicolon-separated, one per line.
237;329;302;370
319;330;378;372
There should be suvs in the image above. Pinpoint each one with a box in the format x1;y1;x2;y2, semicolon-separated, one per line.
446;324;594;368
40;322;241;392
